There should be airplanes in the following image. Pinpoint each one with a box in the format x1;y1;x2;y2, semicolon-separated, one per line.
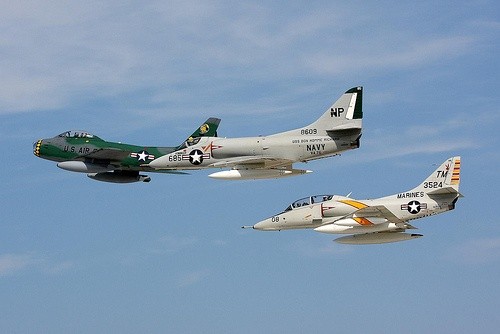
241;155;465;245
139;86;364;180
31;114;224;184
